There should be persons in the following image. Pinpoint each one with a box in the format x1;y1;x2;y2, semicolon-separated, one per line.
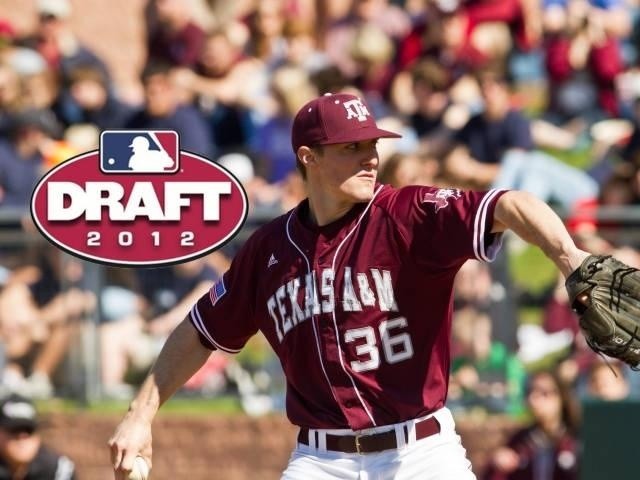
0;1;640;480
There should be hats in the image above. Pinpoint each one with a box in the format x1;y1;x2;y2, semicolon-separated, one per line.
0;396;38;434
291;92;403;152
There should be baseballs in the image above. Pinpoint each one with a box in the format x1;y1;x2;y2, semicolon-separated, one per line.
128;456;149;480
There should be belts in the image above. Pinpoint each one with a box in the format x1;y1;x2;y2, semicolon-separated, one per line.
296;415;441;455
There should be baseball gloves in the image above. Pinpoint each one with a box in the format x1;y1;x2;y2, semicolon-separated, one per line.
565;255;640;370
